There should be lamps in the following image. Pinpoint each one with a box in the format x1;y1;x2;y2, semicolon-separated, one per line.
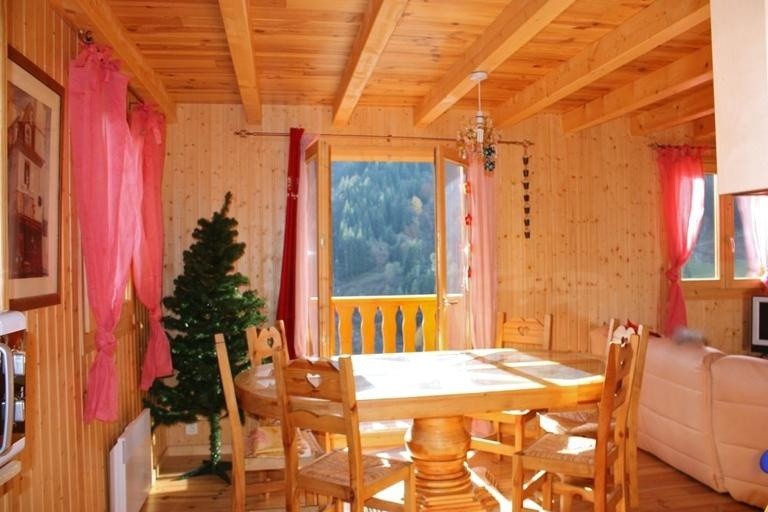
456;72;503;171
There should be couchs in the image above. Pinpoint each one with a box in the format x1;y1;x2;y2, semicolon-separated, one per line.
590;328;767;509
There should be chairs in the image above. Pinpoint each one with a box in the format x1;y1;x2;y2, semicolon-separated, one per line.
243;320;334;505
539;317;649;509
512;334;640;512
214;333;324;512
464;312;552;458
272;351;416;512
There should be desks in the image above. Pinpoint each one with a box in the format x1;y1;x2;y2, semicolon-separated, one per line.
233;348;622;511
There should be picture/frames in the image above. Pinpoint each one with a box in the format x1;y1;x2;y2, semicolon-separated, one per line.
7;43;66;311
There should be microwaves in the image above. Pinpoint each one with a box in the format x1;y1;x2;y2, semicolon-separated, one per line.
0;309;28;468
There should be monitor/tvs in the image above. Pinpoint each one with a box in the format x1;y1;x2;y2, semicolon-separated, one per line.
751;295;768;353
